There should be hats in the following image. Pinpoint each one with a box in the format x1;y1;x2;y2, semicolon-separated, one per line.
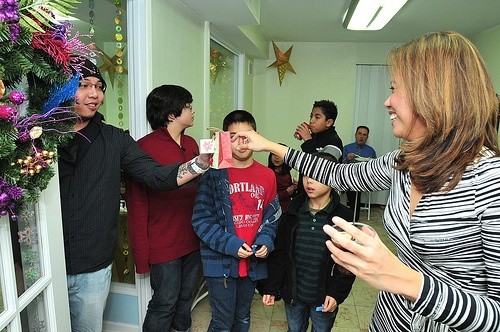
68;55;107;94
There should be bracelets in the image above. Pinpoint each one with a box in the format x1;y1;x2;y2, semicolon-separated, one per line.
190;156;210;175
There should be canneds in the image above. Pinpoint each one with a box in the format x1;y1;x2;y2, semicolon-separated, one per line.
294;122;310;141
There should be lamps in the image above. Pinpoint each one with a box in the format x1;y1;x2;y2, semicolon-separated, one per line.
342;0;408;30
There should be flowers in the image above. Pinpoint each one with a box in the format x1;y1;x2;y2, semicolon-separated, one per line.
0;0;101;221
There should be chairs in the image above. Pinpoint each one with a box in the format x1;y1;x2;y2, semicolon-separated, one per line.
338;191;371;220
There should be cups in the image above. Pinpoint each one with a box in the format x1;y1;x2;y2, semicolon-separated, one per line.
329;222;376;275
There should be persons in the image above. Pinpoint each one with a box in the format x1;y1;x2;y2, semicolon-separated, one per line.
190;109;283;332
296;99;344;196
33;55;215;332
260;151;357;332
323;30;500;332
265;143;298;211
342;126;377;220
122;85;204;331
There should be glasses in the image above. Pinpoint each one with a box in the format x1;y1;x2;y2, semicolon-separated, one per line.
184;105;192;110
78;81;106;92
